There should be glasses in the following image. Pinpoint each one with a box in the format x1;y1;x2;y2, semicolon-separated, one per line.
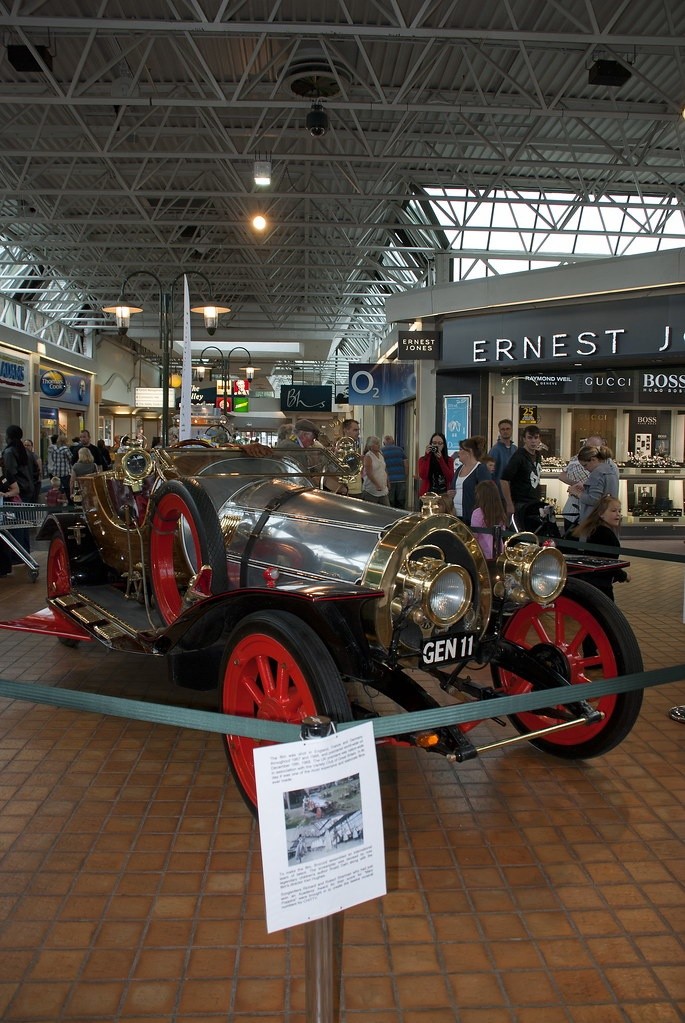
431;441;445;445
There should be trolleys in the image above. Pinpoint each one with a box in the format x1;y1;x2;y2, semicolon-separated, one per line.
0;494;50;582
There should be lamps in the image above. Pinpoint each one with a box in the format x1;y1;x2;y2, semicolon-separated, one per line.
253;148;273;186
584;45;637;86
0;26;57;73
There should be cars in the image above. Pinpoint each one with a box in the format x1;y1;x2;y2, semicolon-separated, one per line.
0;432;646;827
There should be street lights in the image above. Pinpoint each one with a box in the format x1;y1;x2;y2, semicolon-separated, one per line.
196;346;261;433
101;271;231;447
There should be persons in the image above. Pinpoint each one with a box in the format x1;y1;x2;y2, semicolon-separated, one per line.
0;425;178;578
238;419;409;510
416;419;631;671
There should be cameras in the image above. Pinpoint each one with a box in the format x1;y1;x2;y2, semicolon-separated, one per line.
429;446;438;453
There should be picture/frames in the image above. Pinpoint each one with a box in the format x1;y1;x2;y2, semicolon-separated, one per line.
335;384;351;405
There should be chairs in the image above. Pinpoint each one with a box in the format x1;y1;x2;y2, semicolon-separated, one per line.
103;476;163;520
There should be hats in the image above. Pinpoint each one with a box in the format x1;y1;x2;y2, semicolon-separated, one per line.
295;419;321;436
